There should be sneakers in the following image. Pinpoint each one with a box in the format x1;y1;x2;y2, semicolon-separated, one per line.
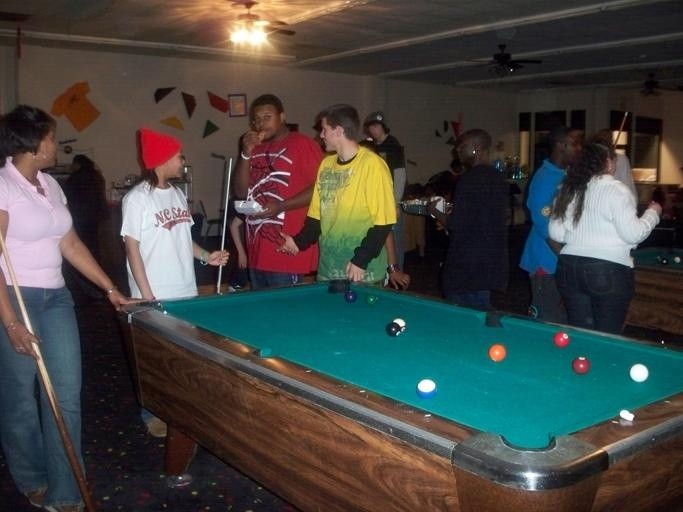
145;417;167;437
24;489;48;507
44;505;88;512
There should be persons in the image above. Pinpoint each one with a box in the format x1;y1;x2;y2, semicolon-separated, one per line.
548;141;664;335
519;123;584;326
119;127;228;437
365;111;406;264
591;128;638;204
385;228;409;291
227;197;250;289
403;182;427;283
0;105;149;512
442;129;511;310
276;104;396;288
228;95;325;290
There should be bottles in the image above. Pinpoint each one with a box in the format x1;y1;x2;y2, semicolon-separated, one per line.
518;169;522;180
512;171;515;179
110;181;116;200
515;170;518;180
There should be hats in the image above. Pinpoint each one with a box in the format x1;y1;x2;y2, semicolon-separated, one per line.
139;127;182;170
363;111;387;125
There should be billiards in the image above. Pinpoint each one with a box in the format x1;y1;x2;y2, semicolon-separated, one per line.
629;363;649;383
386;323;401;335
345;291;357;302
573;356;590;374
661;258;668;265
393;318;406;330
656;255;664;262
366;294;378;304
553;331;570;347
488;343;506;363
673;256;681;264
417;379;436;399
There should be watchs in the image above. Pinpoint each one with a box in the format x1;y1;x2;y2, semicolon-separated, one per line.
240;150;251;161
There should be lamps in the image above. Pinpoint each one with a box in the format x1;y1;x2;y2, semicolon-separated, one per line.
231;20;272;48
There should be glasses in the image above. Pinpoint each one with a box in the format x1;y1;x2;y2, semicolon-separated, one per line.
251;117;270;125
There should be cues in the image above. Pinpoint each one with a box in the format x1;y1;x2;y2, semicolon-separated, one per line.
612;111;629;151
217;157;233;296
1;229;93;512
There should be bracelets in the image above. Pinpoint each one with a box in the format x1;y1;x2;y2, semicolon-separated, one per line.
386;263;400;276
199;249;209;265
104;285;119;295
2;321;17;329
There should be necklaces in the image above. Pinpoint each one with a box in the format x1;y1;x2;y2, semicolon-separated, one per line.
24;174;44;190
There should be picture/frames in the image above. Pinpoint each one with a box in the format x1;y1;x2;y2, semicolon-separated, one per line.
228;93;248;117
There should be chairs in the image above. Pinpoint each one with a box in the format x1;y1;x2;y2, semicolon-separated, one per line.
200;201;225;240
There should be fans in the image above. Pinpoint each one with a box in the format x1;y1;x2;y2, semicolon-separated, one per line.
209;0;295;36
627;74;683;96
468;45;544;76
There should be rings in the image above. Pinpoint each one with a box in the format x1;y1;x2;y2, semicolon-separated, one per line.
16;345;21;351
